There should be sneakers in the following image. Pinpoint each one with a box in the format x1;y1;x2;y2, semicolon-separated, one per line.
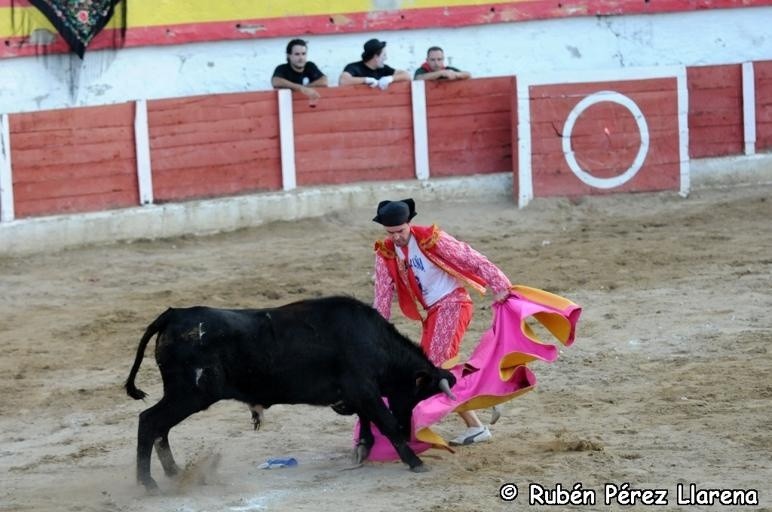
449;426;492;445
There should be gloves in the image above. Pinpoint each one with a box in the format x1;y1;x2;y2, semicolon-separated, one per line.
365;77;379;89
379;75;394;91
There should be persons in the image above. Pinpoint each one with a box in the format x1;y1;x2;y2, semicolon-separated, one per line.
270;39;328;102
370;198;511;446
414;47;471;81
338;40;411;92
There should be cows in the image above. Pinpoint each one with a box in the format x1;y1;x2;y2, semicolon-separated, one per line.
119;291;460;497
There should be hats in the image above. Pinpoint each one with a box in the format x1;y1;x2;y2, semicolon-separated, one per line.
371;198;417;225
362;40;386;60
